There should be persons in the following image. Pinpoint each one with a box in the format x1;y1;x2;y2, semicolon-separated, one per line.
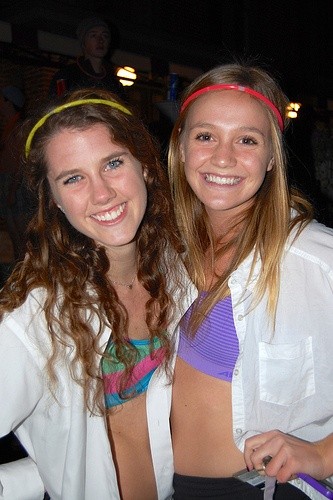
168;65;333;500
0;89;197;500
0;16;190;264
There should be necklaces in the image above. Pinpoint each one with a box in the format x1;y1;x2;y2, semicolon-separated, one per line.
109;271;138;290
5;111;20;130
75;54;107;79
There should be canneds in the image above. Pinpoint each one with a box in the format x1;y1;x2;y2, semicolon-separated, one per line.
56;78;65;97
167;72;179;103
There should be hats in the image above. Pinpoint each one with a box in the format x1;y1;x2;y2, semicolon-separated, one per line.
77;19;109;39
0;86;25;108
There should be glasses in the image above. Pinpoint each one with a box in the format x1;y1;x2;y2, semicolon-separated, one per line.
86;32;111;40
